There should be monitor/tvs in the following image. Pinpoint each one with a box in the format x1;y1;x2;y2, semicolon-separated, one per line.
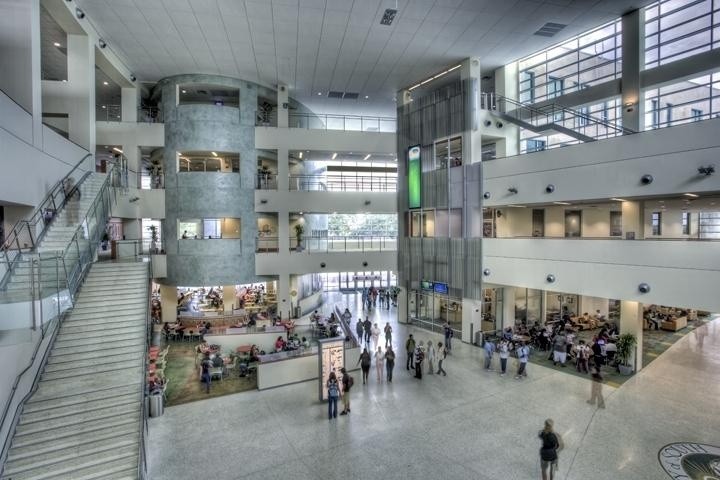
421;281;430;289
434;283;448;293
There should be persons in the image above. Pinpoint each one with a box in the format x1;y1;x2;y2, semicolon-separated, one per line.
538;419;559;480
455;158;461;166
148;289;675;420
182;230;188;239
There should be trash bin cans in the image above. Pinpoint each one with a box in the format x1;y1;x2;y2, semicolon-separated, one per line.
152;332;160;347
476;331;483;347
150;394;163;418
295;307;301;318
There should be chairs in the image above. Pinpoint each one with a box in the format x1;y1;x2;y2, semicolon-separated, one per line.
476;306;710;371
149;245;158;257
152;292;343;404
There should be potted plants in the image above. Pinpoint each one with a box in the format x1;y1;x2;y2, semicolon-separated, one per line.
610;332;637;377
260;100;272;126
294;224;305;252
147;225;157;253
